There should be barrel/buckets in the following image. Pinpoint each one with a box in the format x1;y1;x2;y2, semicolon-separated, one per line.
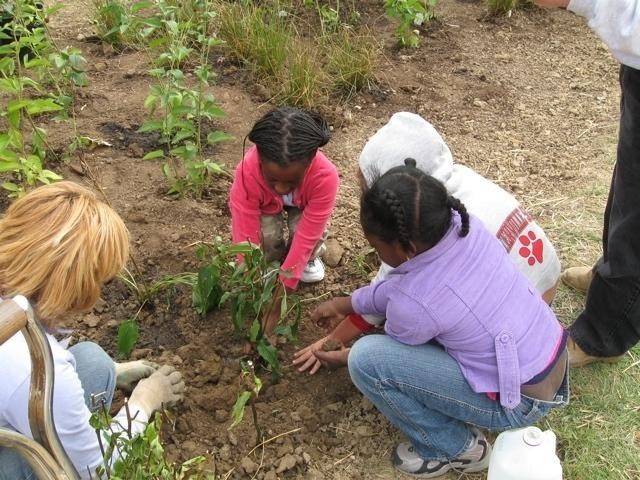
489;425;561;479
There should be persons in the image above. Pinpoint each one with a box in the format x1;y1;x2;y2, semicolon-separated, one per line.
1;181;184;479
292;113;561;378
312;158;570;479
228;108;340;356
528;1;638;364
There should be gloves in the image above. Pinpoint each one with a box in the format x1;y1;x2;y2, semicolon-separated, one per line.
131;365;186;416
115;358;158;395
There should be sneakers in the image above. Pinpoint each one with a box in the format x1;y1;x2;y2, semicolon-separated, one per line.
567;335;623;366
562;267;593;293
300;258;325;283
391;425;493;479
